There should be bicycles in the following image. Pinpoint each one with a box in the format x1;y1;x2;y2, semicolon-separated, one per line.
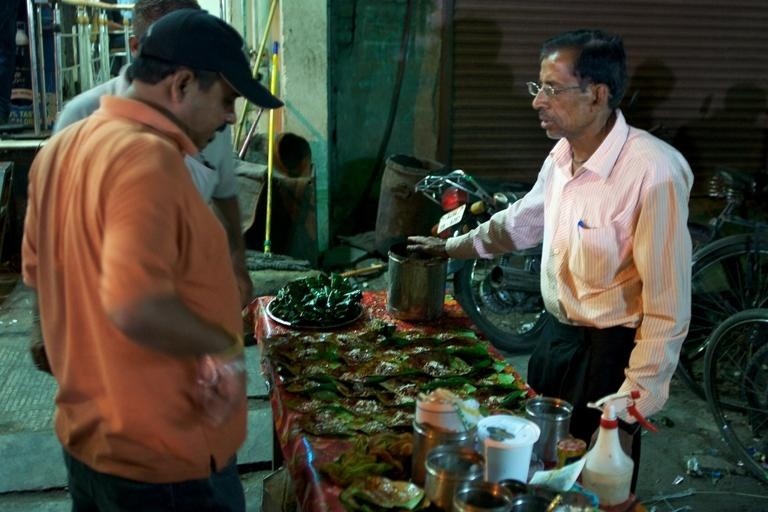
673;196;768;492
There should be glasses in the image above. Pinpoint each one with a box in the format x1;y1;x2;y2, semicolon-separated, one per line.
525;81;581;99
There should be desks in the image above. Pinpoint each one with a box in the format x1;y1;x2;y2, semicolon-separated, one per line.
249;292;649;512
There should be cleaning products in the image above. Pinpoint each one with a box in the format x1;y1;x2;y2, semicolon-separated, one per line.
582;390;655;506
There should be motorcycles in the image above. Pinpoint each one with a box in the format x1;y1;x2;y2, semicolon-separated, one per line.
414;156;768;355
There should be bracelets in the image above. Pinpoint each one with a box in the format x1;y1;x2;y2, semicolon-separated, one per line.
211;329;246;366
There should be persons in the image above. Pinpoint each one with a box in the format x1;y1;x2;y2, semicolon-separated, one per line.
20;9;284;511
29;0;256;378
403;27;697;500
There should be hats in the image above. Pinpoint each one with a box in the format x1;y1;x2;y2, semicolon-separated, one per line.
138;8;284;109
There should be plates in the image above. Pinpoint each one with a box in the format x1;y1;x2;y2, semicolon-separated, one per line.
265;295;364;328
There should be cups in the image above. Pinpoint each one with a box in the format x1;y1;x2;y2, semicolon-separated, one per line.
423;447;487;512
412;421;479;489
451;480;513;512
520;398;574;473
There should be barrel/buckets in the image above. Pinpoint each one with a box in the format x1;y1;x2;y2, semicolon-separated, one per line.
387;249;448;321
375;155;446;263
